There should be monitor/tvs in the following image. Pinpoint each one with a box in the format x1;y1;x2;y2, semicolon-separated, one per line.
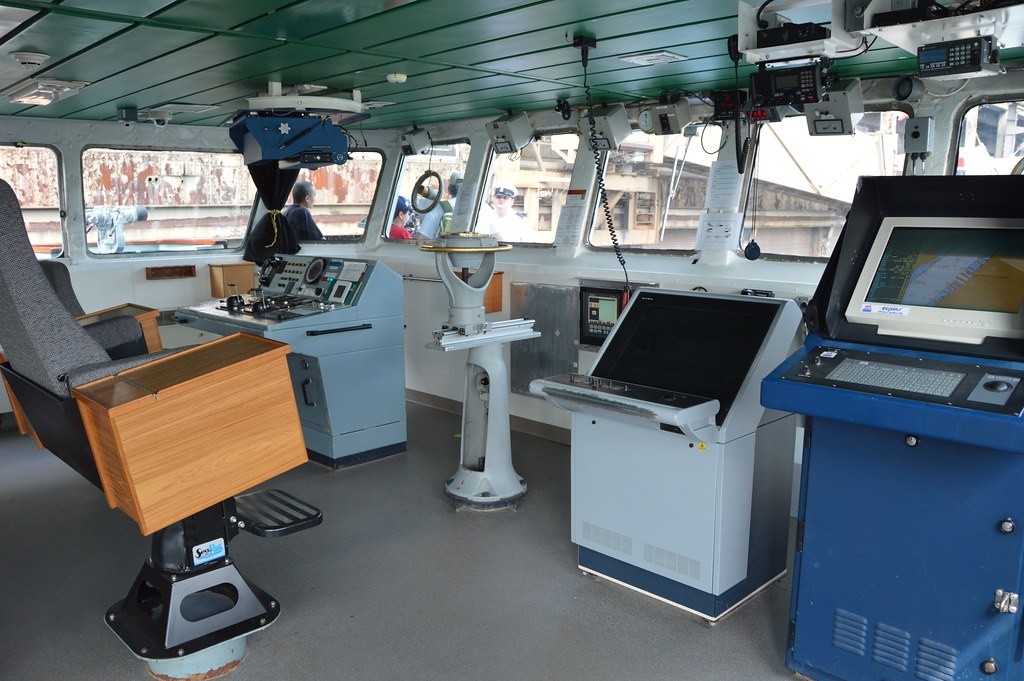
845;217;1024;344
593;290;781;425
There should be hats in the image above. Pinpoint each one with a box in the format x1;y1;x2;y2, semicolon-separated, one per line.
396;196;414;211
449;172;464;185
493;184;517;198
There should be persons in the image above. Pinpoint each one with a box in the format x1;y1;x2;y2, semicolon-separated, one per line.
389;171;535;242
286;180;325;241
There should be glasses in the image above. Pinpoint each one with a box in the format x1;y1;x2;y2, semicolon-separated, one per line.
401;199;411;211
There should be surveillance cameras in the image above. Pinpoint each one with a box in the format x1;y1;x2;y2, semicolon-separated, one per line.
148;111;171;127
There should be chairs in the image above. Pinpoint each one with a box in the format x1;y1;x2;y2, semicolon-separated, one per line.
0;178;323;681
